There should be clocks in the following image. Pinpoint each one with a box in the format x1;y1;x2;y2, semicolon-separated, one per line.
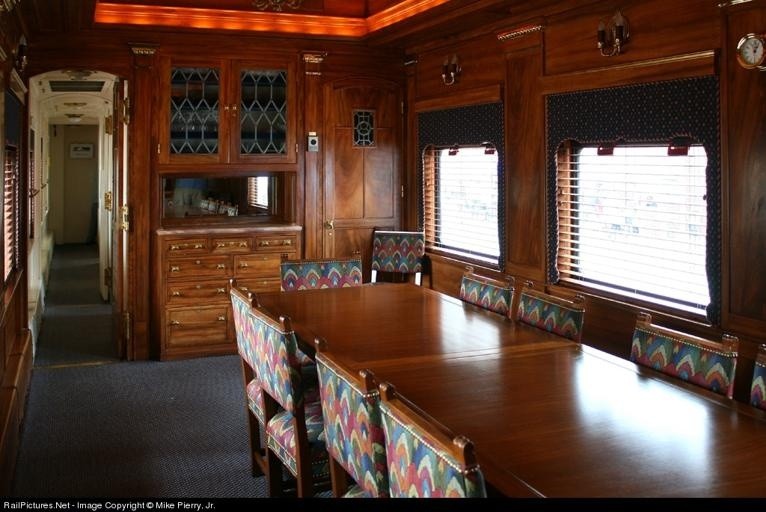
737;32;766;72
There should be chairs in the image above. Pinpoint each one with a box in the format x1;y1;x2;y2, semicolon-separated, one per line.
230;231;763;497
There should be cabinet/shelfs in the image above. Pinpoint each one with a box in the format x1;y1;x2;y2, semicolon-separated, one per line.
150;46;303;361
